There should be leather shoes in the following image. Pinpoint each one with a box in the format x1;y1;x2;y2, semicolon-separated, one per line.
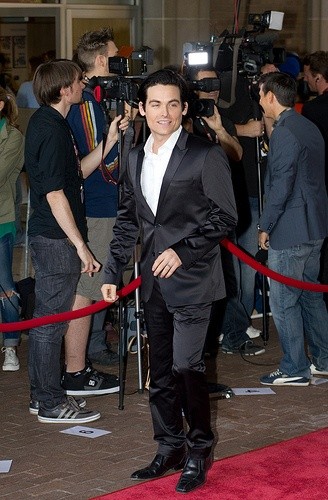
129;454;187;480
176;451;211;493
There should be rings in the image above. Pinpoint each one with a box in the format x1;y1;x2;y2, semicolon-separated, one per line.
167;264;171;268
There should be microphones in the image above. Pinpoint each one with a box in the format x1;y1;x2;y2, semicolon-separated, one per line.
245;32;295;44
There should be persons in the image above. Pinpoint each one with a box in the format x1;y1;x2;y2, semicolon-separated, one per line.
0;51;328;371
24;58;102;423
101;69;239;492
61;25;138;396
258;71;328;385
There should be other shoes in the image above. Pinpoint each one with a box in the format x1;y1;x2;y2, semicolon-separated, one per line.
251;309;264;319
219;334;224;344
246;326;262;338
89;349;120;365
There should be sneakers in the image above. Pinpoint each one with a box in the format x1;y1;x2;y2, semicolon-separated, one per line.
259;369;310;387
62;366;120;396
37;396;102;423
29;393;87;416
1;346;20;371
220;333;266;356
61;359;119;380
309;360;328;375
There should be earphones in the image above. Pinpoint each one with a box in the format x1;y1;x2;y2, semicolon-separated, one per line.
316;78;319;81
